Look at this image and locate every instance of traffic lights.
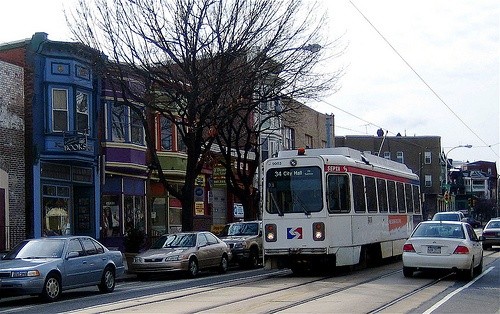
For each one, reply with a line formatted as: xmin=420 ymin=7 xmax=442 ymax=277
xmin=444 ymin=193 xmax=449 ymax=204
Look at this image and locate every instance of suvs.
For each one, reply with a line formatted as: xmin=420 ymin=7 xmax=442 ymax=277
xmin=427 ymin=211 xmax=466 ymax=222
xmin=217 ymin=220 xmax=263 ymax=268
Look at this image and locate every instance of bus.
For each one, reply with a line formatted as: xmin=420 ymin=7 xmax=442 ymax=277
xmin=260 ymin=132 xmax=424 ymax=276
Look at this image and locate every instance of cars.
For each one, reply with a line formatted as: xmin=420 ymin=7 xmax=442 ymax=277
xmin=482 ymin=219 xmax=500 ymax=250
xmin=465 ymin=217 xmax=482 ymax=229
xmin=402 ymin=221 xmax=486 ymax=281
xmin=131 ymin=231 xmax=232 ymax=281
xmin=0 ymin=235 xmax=126 ymax=303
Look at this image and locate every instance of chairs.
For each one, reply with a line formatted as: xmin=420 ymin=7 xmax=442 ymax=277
xmin=429 ymin=228 xmax=439 ymax=235
xmin=452 ymin=230 xmax=461 ymax=237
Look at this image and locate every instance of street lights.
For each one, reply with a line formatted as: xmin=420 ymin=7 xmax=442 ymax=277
xmin=445 ymin=144 xmax=473 ymax=211
xmin=251 ymin=44 xmax=323 ymax=189
